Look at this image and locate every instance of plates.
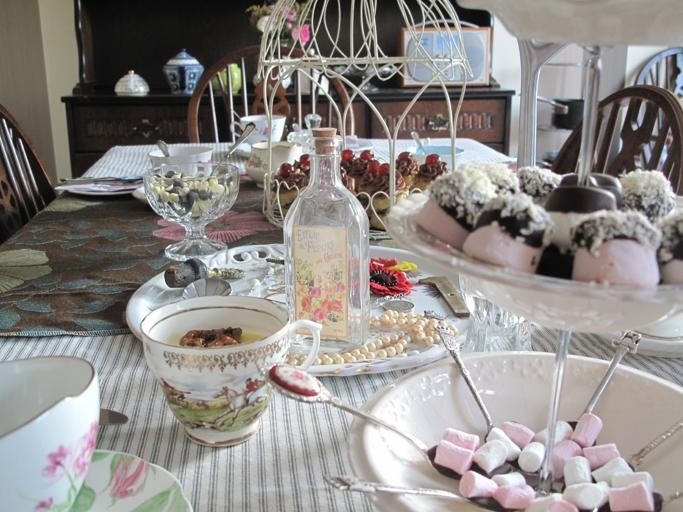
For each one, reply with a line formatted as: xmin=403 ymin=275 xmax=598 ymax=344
xmin=132 ymin=187 xmax=146 ymax=202
xmin=62 ymin=189 xmax=128 ymax=199
xmin=73 ymin=449 xmax=194 ymax=512
xmin=119 ymin=241 xmax=471 ymax=380
xmin=344 ymin=138 xmax=372 ymax=154
xmin=226 ymin=144 xmax=252 ymax=156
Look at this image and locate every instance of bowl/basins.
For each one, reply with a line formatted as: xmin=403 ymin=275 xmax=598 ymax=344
xmin=0 ymin=354 xmax=103 ymax=512
xmin=407 ymin=145 xmax=466 ymax=169
xmin=337 ymin=350 xmax=682 ymax=511
xmin=245 ymin=141 xmax=303 ymax=190
xmin=386 ymin=186 xmax=683 ymax=332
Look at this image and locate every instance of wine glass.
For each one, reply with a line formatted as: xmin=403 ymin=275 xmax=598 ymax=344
xmin=141 ymin=160 xmax=244 ymax=259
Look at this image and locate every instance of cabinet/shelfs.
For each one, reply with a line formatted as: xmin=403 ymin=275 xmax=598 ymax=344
xmin=59 ymin=93 xmax=214 ymax=177
xmin=214 ymin=93 xmax=370 ymax=141
xmin=370 ymin=90 xmax=518 ymax=157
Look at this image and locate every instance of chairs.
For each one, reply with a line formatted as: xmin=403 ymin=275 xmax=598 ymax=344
xmin=630 ymin=44 xmax=683 ymax=172
xmin=186 ymin=40 xmax=357 ymax=145
xmin=0 ymin=103 xmax=57 ymax=241
xmin=549 ymin=83 xmax=683 ymax=201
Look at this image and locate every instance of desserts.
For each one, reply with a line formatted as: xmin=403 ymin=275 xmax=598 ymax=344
xmin=268 ymin=149 xmax=683 ymax=287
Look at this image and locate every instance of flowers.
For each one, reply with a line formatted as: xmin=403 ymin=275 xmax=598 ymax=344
xmin=249 ymin=0 xmax=320 ymax=59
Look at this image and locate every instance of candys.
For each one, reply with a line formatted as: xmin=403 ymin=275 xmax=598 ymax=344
xmin=434 ymin=413 xmax=656 ymax=512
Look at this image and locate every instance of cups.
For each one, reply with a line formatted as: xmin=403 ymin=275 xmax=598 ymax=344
xmin=227 ymin=114 xmax=287 ymax=144
xmin=139 ymin=295 xmax=321 ymax=448
xmin=147 ymin=147 xmax=214 ymax=173
xmin=212 ymin=62 xmax=243 ymax=95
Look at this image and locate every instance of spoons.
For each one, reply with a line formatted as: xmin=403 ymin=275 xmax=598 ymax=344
xmin=434 ymin=326 xmax=541 ymax=476
xmin=257 ymin=365 xmax=484 ymax=479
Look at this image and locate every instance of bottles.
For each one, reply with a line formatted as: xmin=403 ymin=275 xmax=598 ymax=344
xmin=114 ymin=69 xmax=152 ymax=97
xmin=163 ymin=48 xmax=204 ymax=95
xmin=296 ymin=48 xmax=330 ymax=95
xmin=282 ymin=126 xmax=374 ymax=348
